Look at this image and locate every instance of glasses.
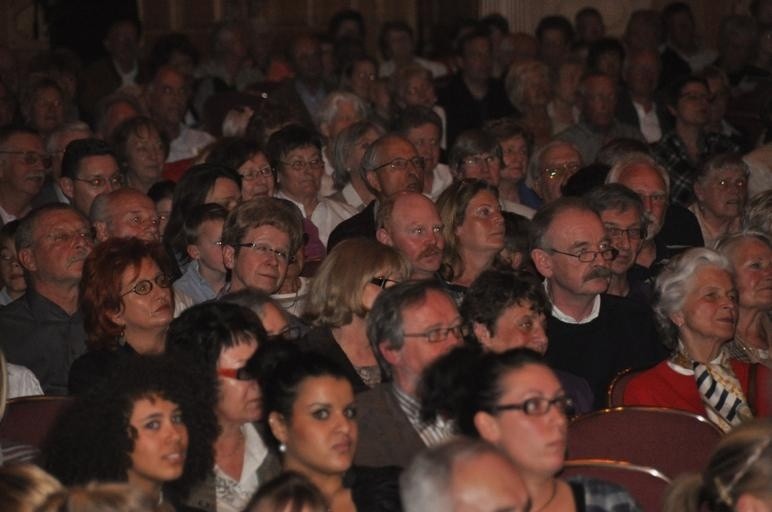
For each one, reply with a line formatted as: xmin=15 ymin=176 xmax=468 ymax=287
xmin=605 ymin=226 xmax=646 ymax=240
xmin=373 ymin=157 xmax=423 ymax=172
xmin=75 ymin=174 xmax=123 ymax=186
xmin=279 ymin=159 xmax=324 ymax=172
xmin=552 ymin=246 xmax=616 ymax=261
xmin=369 ymin=277 xmax=398 ymax=289
xmin=120 ymin=274 xmax=171 ymax=297
xmin=241 ymin=243 xmax=289 ymax=259
xmin=404 ymin=325 xmax=469 ymax=342
xmin=235 ymin=168 xmax=274 ymax=180
xmin=1 ymin=150 xmax=52 ymax=168
xmin=498 ymin=395 xmax=573 ymax=415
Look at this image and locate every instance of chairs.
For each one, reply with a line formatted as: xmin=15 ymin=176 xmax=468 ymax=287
xmin=605 ymin=364 xmax=656 ymax=405
xmin=561 ymin=404 xmax=725 ymax=487
xmin=2 ymin=392 xmax=82 ymax=465
xmin=553 ymin=457 xmax=676 ymax=512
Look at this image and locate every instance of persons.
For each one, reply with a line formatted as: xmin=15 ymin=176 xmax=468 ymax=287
xmin=0 ymin=1 xmax=772 ymax=511
